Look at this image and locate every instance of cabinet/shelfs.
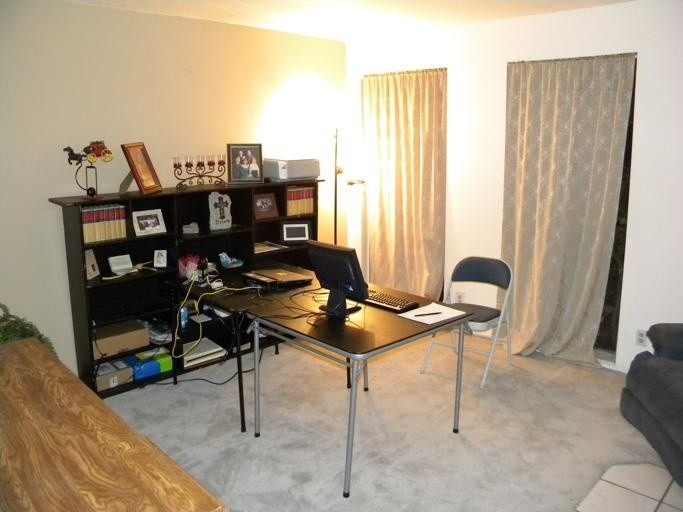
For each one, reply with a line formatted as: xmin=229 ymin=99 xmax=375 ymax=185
xmin=175 ymin=186 xmax=253 ymax=376
xmin=78 ymin=193 xmax=178 ymax=397
xmin=252 ymin=180 xmax=318 ymax=350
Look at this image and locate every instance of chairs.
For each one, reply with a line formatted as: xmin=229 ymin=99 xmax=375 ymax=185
xmin=420 ymin=255 xmax=515 ymax=391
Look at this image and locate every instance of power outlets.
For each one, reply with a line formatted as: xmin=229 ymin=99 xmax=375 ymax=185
xmin=635 ymin=328 xmax=647 ymax=347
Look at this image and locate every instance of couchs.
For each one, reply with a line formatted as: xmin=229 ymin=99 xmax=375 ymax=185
xmin=618 ymin=322 xmax=683 ymax=490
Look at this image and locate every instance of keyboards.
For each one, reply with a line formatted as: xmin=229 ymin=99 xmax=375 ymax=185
xmin=363 ymin=289 xmax=419 ymax=313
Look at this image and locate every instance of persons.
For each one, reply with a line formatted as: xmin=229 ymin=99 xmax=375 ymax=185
xmin=235 ymin=149 xmax=260 ymax=179
xmin=157 ymin=252 xmax=165 ymax=265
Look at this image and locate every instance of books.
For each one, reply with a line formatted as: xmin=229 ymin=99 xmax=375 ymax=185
xmin=82 ymin=206 xmax=129 ymax=244
xmin=286 ymin=187 xmax=315 ymax=215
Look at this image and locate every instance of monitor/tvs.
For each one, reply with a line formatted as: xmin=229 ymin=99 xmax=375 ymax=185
xmin=305 ymin=238 xmax=369 ymax=318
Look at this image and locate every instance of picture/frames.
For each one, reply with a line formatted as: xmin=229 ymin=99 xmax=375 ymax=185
xmin=251 ymin=192 xmax=280 ymax=220
xmin=226 ymin=143 xmax=264 ymax=185
xmin=279 ymin=221 xmax=313 ymax=245
xmin=132 ymin=208 xmax=168 ymax=237
xmin=120 ymin=142 xmax=163 ymax=197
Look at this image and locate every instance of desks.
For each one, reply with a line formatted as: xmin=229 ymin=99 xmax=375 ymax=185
xmin=170 ymin=259 xmax=328 ymax=434
xmin=245 ymin=281 xmax=476 ymax=498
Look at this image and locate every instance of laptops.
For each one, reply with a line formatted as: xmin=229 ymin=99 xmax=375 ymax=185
xmin=251 ymin=267 xmax=313 ymax=291
